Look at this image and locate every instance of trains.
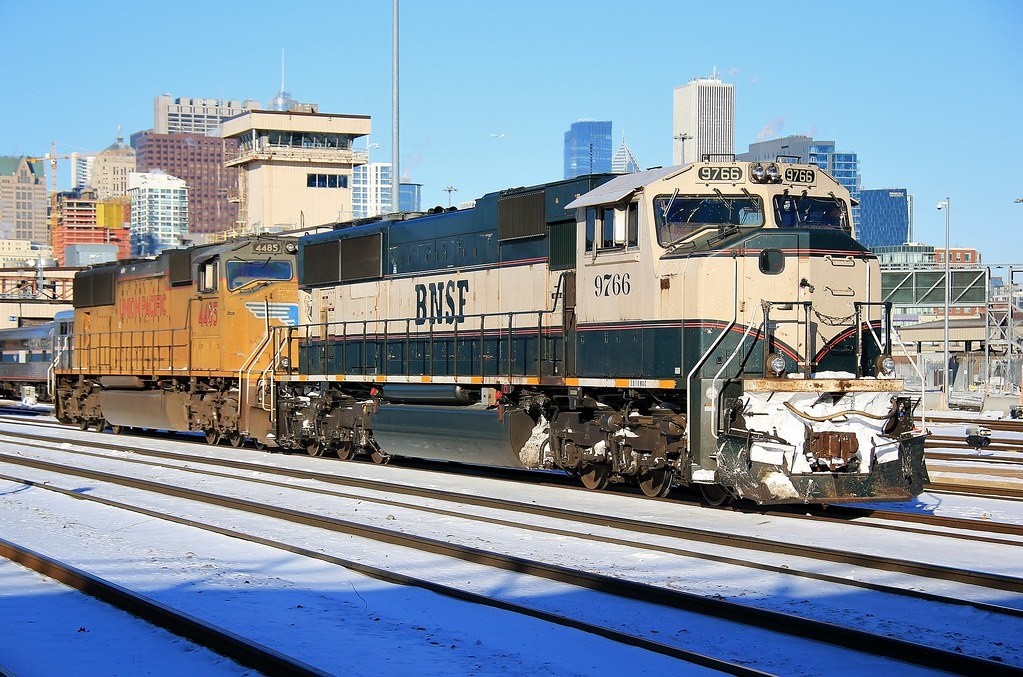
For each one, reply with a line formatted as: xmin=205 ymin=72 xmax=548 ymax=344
xmin=46 ymin=149 xmax=934 ymax=523
xmin=0 ymin=308 xmax=75 ymax=403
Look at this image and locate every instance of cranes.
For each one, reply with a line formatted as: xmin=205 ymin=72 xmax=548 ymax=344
xmin=24 ymin=142 xmax=136 ymax=267
